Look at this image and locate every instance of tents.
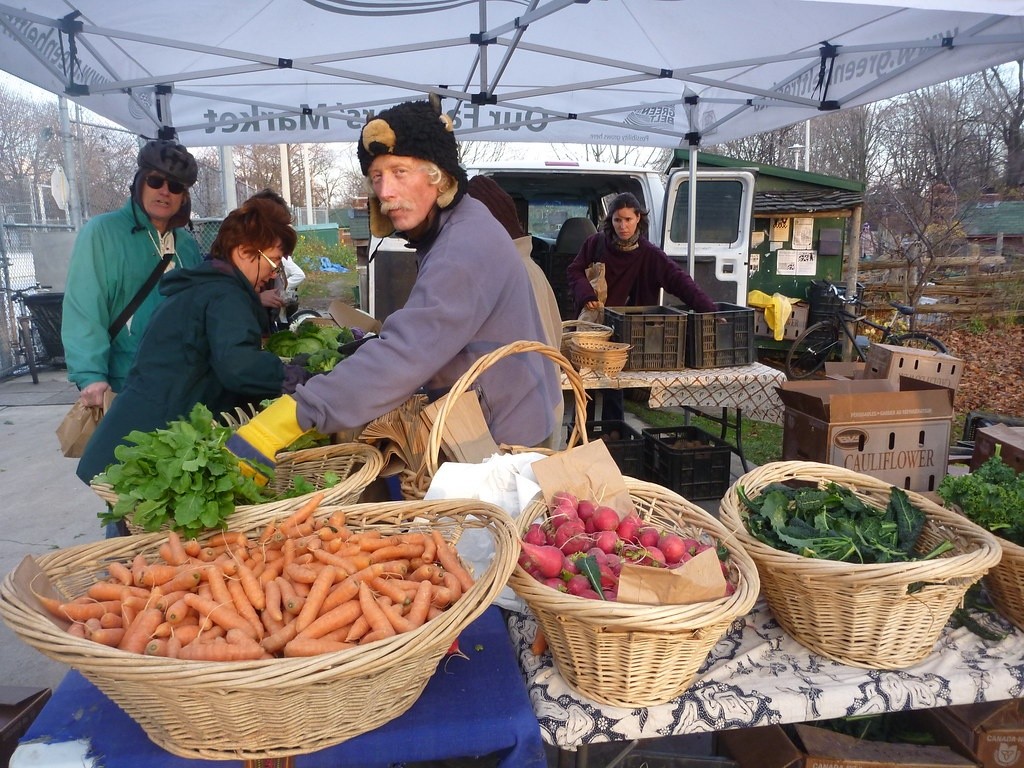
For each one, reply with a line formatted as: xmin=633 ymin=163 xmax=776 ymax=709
xmin=0 ymin=0 xmax=1024 ymax=317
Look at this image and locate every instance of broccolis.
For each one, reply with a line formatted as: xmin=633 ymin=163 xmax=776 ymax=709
xmin=302 ymin=349 xmax=343 ymax=371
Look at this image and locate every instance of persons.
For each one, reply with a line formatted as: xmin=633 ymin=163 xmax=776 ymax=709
xmin=567 ymin=193 xmax=727 ymax=432
xmin=61 ymin=101 xmax=564 ymax=576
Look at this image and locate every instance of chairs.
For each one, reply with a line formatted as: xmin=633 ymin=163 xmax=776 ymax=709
xmin=556 ymin=217 xmax=599 ymax=252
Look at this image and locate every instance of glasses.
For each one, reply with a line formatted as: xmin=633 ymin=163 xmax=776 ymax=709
xmin=257 ymin=248 xmax=281 ymax=277
xmin=144 ymin=176 xmax=185 ymax=195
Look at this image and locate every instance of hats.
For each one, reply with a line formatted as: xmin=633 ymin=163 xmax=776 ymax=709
xmin=465 ymin=176 xmax=524 ymax=239
xmin=357 ymin=100 xmax=467 ymax=209
xmin=137 ymin=141 xmax=198 ymax=186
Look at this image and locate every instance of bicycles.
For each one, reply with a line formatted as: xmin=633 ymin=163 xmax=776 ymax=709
xmin=0 ymin=282 xmax=54 ymax=385
xmin=785 ymin=277 xmax=950 ymax=383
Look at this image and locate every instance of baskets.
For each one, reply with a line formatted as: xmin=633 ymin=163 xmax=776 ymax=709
xmin=3 ymin=462 xmax=1024 ymax=760
xmin=91 ymin=442 xmax=382 ymax=536
xmin=400 ymin=344 xmax=589 ymax=502
xmin=560 ymin=319 xmax=634 ymax=377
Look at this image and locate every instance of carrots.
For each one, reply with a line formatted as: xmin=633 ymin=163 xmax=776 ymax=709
xmin=532 ymin=625 xmax=549 ymax=655
xmin=28 ymin=492 xmax=477 ymax=662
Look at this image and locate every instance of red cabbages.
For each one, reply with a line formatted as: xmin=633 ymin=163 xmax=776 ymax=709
xmin=348 ymin=326 xmax=365 ymax=341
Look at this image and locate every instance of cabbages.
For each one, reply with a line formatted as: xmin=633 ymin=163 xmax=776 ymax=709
xmin=267 ymin=319 xmax=355 ymax=357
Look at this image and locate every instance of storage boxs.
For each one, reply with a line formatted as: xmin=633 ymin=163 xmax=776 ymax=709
xmin=807 ymin=280 xmax=865 ymax=340
xmin=349 ymin=192 xmax=370 ymax=240
xmin=304 ymin=300 xmax=383 ymax=335
xmin=669 ymin=301 xmax=756 ymax=369
xmin=969 ymin=423 xmax=1024 ymax=477
xmin=749 ymin=301 xmax=809 ymax=340
xmin=772 ymin=343 xmax=967 ymax=505
xmin=642 ymin=425 xmax=731 ymax=500
xmin=957 ymin=411 xmax=1024 ymax=448
xmin=712 ymin=698 xmax=1023 ymax=768
xmin=530 ymin=250 xmax=578 ymax=303
xmin=0 ymin=685 xmax=52 ymax=767
xmin=566 ymin=419 xmax=644 ymax=477
xmin=604 ymin=306 xmax=688 ymax=372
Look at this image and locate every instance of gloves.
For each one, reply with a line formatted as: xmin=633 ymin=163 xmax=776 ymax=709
xmin=225 ymin=397 xmax=314 ymax=485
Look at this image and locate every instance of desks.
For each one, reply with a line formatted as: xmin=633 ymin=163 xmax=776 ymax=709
xmin=8 ymin=605 xmax=551 ymax=767
xmin=560 ymin=361 xmax=788 ymax=474
xmin=507 ymin=589 xmax=1023 ymax=768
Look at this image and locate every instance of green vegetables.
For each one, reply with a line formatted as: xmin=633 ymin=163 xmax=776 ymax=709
xmin=935 ymin=442 xmax=1024 ymax=550
xmin=737 ymin=481 xmax=954 ymax=594
xmin=86 ymin=400 xmax=341 ymax=541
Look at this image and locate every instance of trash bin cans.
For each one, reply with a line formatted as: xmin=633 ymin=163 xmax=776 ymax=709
xmin=25 ymin=291 xmax=64 ymax=368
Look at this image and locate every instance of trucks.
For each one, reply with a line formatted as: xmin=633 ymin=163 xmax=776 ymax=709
xmin=365 ymin=158 xmax=760 ymax=325
